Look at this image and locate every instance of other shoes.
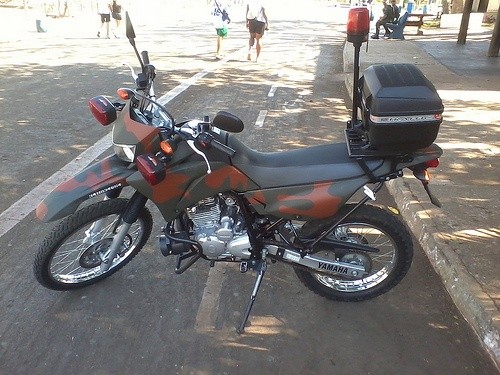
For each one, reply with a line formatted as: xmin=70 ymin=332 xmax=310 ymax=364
xmin=384 ymin=32 xmax=391 ymax=37
xmin=97 ymin=31 xmax=100 ymax=37
xmin=371 ymin=35 xmax=379 ymax=39
xmin=216 ymin=55 xmax=221 ymax=60
xmin=247 ymin=52 xmax=252 ymax=60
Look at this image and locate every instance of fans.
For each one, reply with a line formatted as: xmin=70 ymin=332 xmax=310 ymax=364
xmin=356 ymin=63 xmax=445 ymax=151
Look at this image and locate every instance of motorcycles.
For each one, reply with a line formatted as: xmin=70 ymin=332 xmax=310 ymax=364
xmin=32 ymin=10 xmax=445 ymax=335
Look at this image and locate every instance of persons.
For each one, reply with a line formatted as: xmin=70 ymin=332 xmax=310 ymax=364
xmin=245 ymin=0 xmax=269 ymax=62
xmin=96 ymin=0 xmax=113 ymax=39
xmin=210 ymin=0 xmax=230 ymax=60
xmin=111 ymin=0 xmax=123 ymax=39
xmin=370 ymin=0 xmax=400 ymax=39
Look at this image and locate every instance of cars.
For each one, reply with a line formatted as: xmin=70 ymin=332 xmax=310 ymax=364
xmin=328 ymin=0 xmax=375 ymax=21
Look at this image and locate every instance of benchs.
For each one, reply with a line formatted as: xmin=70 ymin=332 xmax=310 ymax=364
xmin=386 ymin=12 xmax=409 ymax=40
